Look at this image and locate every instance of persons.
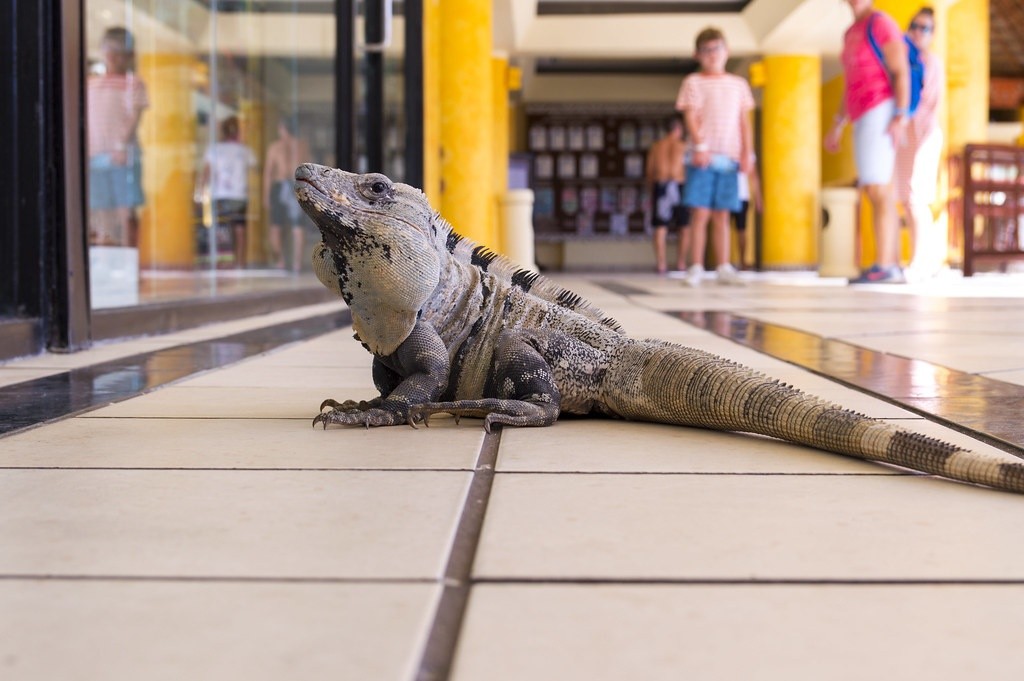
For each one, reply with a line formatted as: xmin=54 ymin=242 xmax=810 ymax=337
xmin=645 ymin=118 xmax=764 ymax=279
xmin=676 ymin=29 xmax=759 ymax=286
xmin=85 ymin=26 xmax=146 ymax=245
xmin=194 ymin=115 xmax=319 ymax=273
xmin=822 ymin=0 xmax=941 ymax=286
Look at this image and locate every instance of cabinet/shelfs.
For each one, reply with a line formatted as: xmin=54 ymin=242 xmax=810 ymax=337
xmin=947 ymin=143 xmax=1024 ymax=276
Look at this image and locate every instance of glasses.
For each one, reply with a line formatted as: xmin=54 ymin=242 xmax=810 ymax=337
xmin=909 ymin=21 xmax=934 ymax=35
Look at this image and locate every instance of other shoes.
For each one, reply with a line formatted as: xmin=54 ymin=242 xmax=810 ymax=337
xmin=717 ymin=264 xmax=748 ymax=286
xmin=683 ymin=264 xmax=704 ymax=287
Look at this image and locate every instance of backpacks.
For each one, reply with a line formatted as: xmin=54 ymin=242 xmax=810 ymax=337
xmin=865 ymin=13 xmax=923 ymax=114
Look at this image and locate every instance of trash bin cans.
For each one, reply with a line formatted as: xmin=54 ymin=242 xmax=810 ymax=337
xmin=818 ymin=187 xmax=858 ymax=278
xmin=506 ymin=188 xmax=540 ymax=277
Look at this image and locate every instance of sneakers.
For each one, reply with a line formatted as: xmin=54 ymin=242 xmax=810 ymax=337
xmin=848 ymin=264 xmax=907 ymax=284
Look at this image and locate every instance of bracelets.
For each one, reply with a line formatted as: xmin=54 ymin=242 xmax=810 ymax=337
xmin=834 ymin=113 xmax=848 ymax=126
xmin=690 ymin=142 xmax=708 ymax=152
xmin=112 ymin=138 xmax=128 ymax=152
xmin=744 ymin=150 xmax=757 ymax=163
